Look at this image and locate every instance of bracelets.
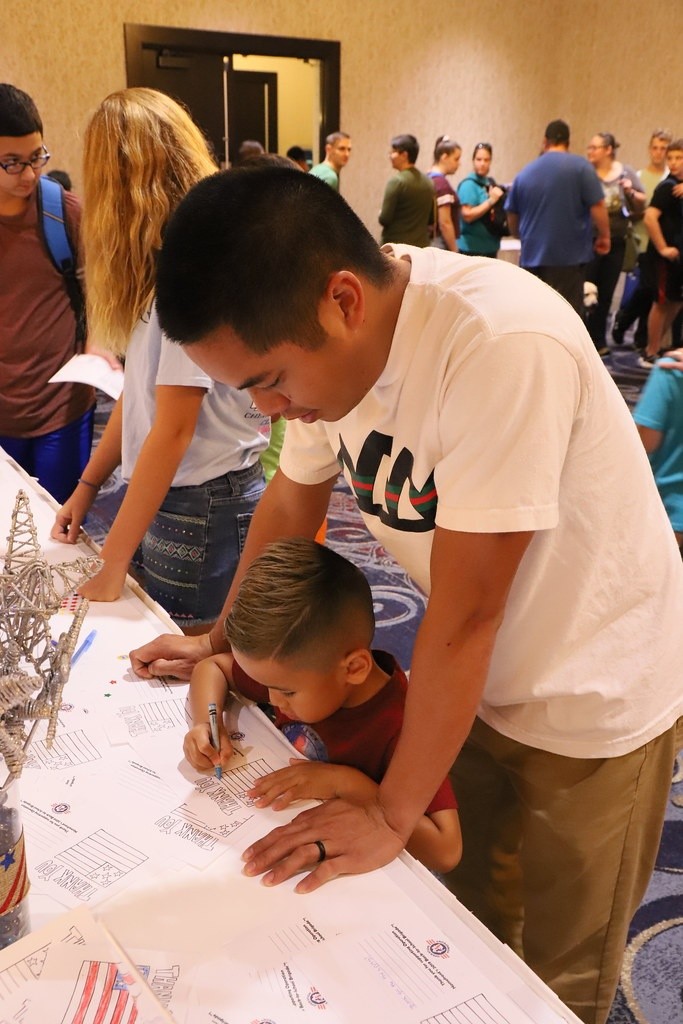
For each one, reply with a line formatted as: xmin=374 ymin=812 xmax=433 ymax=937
xmin=78 ymin=476 xmax=102 ymax=494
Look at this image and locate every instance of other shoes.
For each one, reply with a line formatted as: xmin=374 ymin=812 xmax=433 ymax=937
xmin=597 ymin=346 xmax=611 ymax=359
xmin=638 ymin=347 xmax=668 ymax=368
xmin=612 ymin=311 xmax=625 ymax=343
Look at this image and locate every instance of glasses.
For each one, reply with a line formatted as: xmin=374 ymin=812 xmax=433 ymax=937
xmin=0 ymin=146 xmax=51 ymax=175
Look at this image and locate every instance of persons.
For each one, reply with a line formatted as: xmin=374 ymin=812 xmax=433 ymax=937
xmin=633 ymin=352 xmax=683 ymax=546
xmin=131 ymin=164 xmax=681 ymax=1021
xmin=234 ymin=115 xmax=682 ymax=372
xmin=183 ymin=535 xmax=463 ymax=876
xmin=52 ymin=88 xmax=267 ymax=630
xmin=1 ymin=83 xmax=94 ymax=510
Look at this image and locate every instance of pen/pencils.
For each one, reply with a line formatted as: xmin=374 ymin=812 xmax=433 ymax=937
xmin=70 ymin=629 xmax=98 ymax=666
xmin=208 ymin=703 xmax=221 ymax=780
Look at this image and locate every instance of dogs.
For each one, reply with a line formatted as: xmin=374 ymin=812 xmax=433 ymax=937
xmin=583 ymin=282 xmax=599 ymax=317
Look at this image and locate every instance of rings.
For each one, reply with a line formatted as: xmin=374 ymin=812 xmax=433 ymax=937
xmin=312 ymin=840 xmax=327 ymax=864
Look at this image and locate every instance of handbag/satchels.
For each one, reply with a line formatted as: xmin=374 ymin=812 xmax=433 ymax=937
xmin=621 ymin=226 xmax=642 ymax=272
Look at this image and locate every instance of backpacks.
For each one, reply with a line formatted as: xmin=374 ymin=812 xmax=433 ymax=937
xmin=458 ymin=177 xmax=511 ymax=237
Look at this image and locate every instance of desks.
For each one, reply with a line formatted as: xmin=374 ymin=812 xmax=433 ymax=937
xmin=0 ymin=445 xmax=583 ymax=1024
